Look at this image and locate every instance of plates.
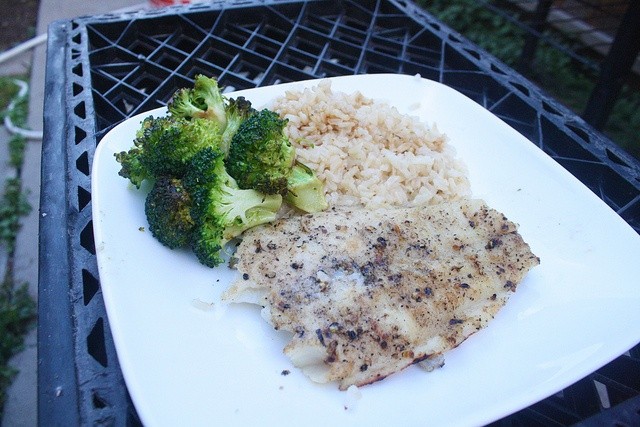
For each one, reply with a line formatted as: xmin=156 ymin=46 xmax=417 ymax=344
xmin=91 ymin=73 xmax=640 ymax=427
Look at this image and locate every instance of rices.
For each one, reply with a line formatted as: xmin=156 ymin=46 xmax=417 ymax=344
xmin=269 ymin=80 xmax=470 ymax=206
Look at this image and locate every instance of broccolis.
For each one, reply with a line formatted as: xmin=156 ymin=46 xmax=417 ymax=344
xmin=113 ymin=73 xmax=326 ymax=270
